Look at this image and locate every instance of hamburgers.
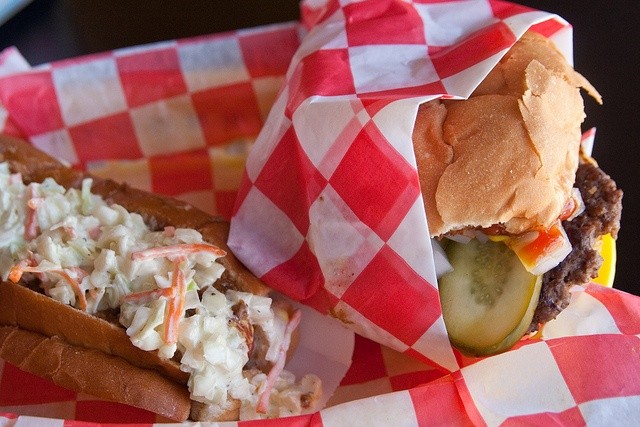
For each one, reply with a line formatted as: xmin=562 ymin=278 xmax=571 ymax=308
xmin=412 ymin=30 xmax=622 ymax=359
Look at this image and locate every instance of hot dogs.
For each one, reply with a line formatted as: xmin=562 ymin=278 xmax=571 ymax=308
xmin=2 ymin=131 xmax=298 ymax=422
